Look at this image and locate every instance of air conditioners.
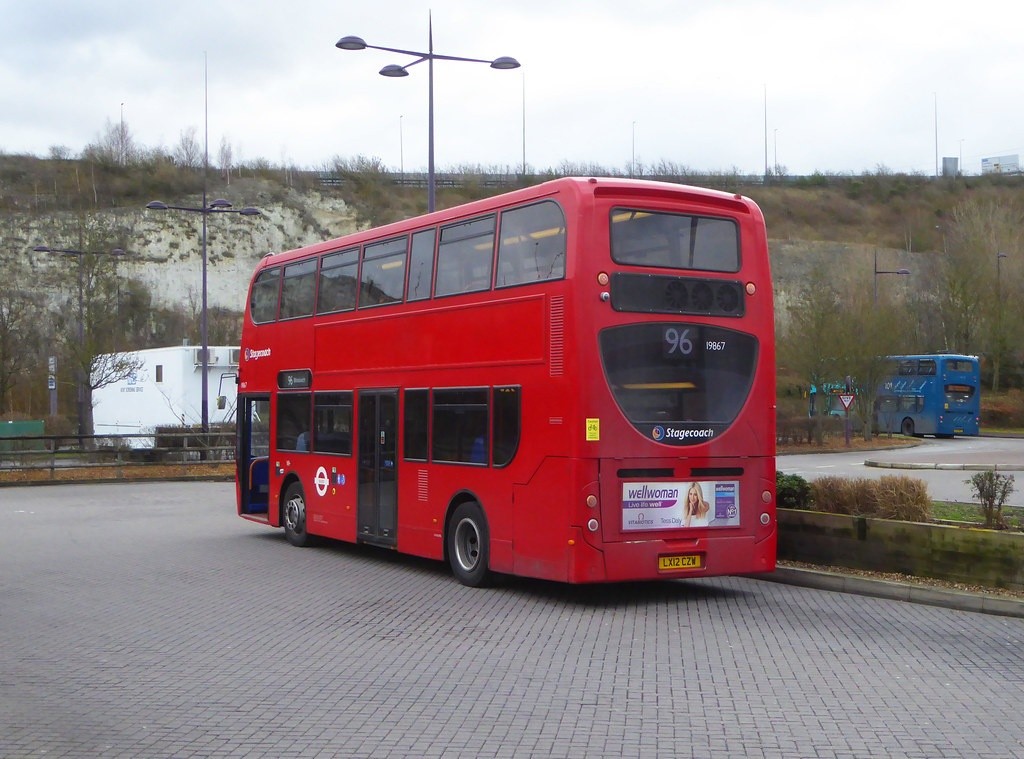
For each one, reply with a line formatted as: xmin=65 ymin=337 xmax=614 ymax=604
xmin=228 ymin=348 xmax=241 ymax=365
xmin=193 ymin=347 xmax=216 ymax=365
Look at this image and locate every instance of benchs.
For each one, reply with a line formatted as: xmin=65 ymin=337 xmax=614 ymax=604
xmin=154 ymin=427 xmax=221 ymax=460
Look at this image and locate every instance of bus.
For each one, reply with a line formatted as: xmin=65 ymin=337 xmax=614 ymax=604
xmin=216 ymin=176 xmax=779 ymax=594
xmin=807 ymin=352 xmax=983 ymax=438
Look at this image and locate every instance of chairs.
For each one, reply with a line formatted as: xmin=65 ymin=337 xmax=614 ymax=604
xmin=296 ymin=431 xmax=311 ymax=451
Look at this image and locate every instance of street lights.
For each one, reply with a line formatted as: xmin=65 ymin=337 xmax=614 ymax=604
xmin=144 ymin=187 xmax=261 ymax=461
xmin=119 ymin=101 xmax=125 ymax=165
xmin=872 ymin=247 xmax=911 ymax=306
xmin=996 ymin=249 xmax=1007 ymax=296
xmin=632 ymin=120 xmax=635 ymax=178
xmin=334 ymin=8 xmax=524 ymax=213
xmin=400 ymin=114 xmax=405 ymax=184
xmin=33 ymin=226 xmax=130 ymax=450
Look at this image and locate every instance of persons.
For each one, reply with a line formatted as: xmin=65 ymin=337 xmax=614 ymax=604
xmin=682 ymin=482 xmax=710 ymax=527
xmin=296 ymin=419 xmax=311 ymax=452
xmin=471 ymin=424 xmax=494 ymax=464
